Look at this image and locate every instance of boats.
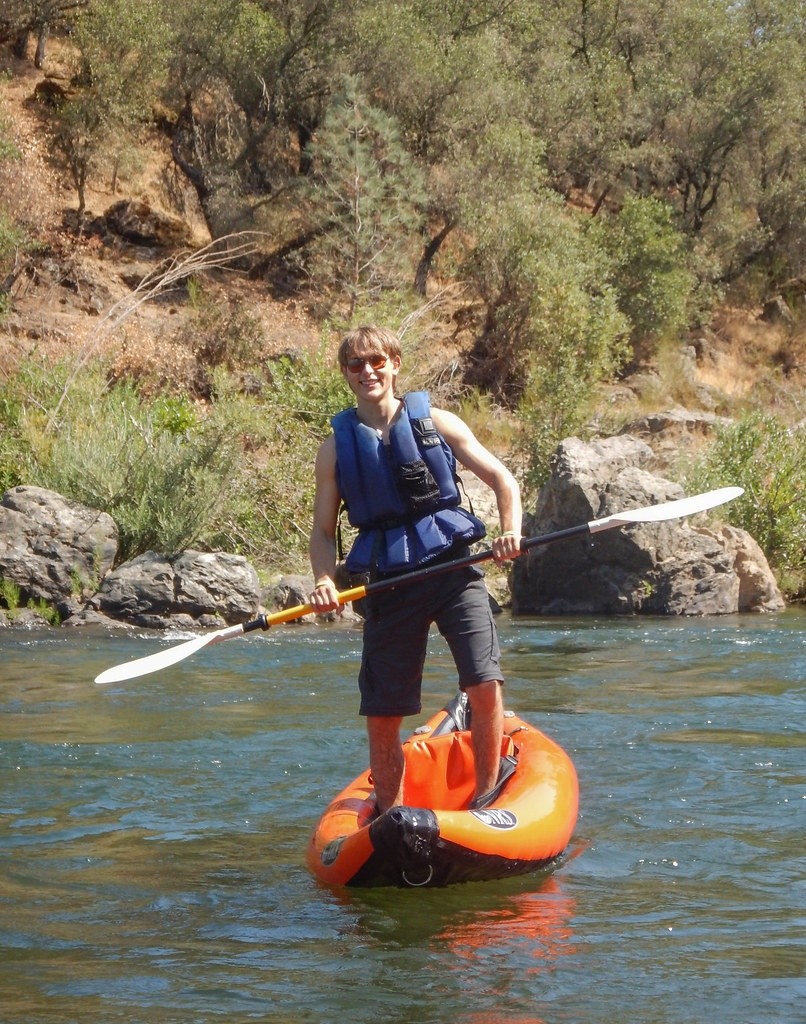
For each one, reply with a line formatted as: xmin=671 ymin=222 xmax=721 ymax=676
xmin=305 ymin=685 xmax=580 ymax=889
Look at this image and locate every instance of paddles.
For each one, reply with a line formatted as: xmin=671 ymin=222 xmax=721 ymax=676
xmin=92 ymin=486 xmax=745 ymax=687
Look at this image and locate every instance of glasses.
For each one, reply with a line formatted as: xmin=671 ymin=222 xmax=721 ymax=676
xmin=343 ymin=355 xmax=391 ymax=373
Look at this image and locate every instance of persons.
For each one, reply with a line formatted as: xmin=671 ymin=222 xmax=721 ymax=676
xmin=309 ymin=326 xmax=523 ymax=816
